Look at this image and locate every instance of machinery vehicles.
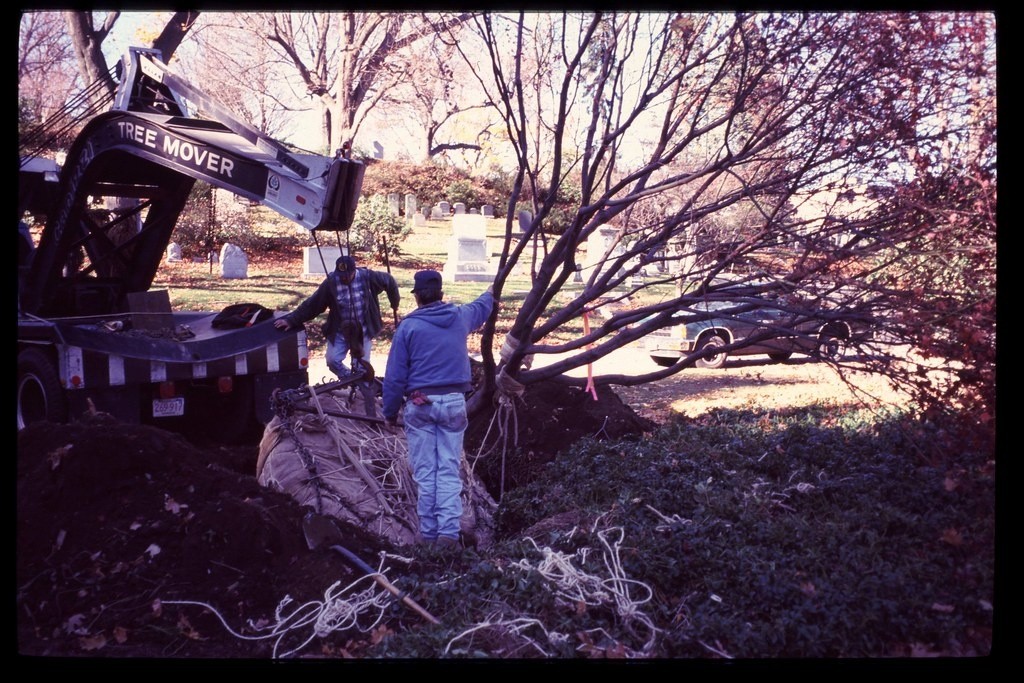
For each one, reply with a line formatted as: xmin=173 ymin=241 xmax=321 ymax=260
xmin=21 ymin=45 xmax=368 ymax=452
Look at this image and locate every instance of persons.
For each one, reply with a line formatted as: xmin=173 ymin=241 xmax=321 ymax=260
xmin=385 ymin=269 xmax=499 ymax=555
xmin=274 ymin=257 xmax=400 ymax=385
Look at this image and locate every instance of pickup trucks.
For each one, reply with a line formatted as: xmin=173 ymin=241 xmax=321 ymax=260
xmin=646 ymin=279 xmax=876 ymax=368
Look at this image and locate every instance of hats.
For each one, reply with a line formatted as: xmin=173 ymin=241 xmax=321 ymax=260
xmin=410 ymin=270 xmax=442 ymax=293
xmin=334 ymin=256 xmax=354 ymax=272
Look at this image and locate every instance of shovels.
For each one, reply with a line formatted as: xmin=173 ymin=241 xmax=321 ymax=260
xmin=302 ymin=512 xmax=441 ymax=625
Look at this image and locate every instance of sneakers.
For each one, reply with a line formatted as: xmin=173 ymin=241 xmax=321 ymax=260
xmin=428 ymin=538 xmax=462 ymax=554
